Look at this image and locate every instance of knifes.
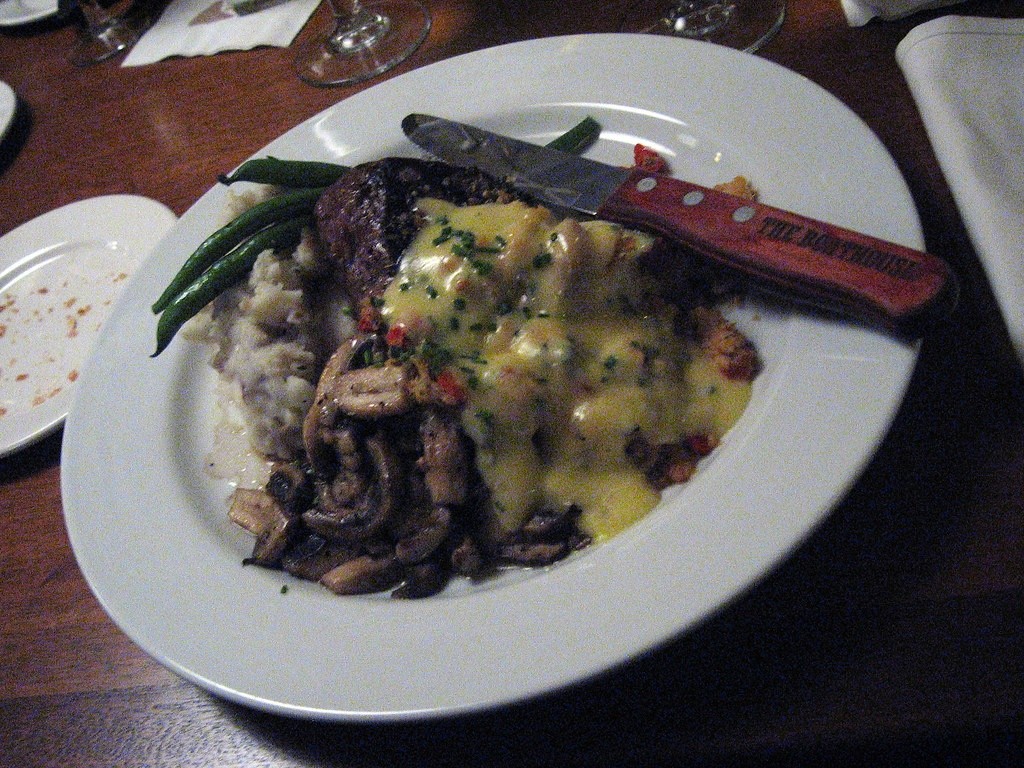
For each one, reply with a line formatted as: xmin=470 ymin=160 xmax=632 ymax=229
xmin=190 ymin=1 xmax=288 ymax=26
xmin=402 ymin=113 xmax=957 ymax=335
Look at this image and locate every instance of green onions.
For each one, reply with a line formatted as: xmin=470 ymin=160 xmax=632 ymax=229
xmin=336 ymin=217 xmax=617 ymax=432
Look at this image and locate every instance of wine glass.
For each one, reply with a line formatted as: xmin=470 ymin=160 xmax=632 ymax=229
xmin=293 ymin=0 xmax=430 ymax=87
xmin=56 ymin=1 xmax=149 ymax=69
xmin=617 ymin=2 xmax=786 ymax=53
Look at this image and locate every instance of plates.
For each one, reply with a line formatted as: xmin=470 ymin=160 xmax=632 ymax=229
xmin=1 ymin=1 xmax=57 ymax=26
xmin=1 ymin=194 xmax=178 ymax=459
xmin=60 ymin=32 xmax=925 ymax=722
xmin=1 ymin=82 xmax=18 ymax=141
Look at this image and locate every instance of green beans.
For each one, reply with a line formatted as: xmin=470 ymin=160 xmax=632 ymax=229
xmin=148 ymin=118 xmax=598 ymax=358
